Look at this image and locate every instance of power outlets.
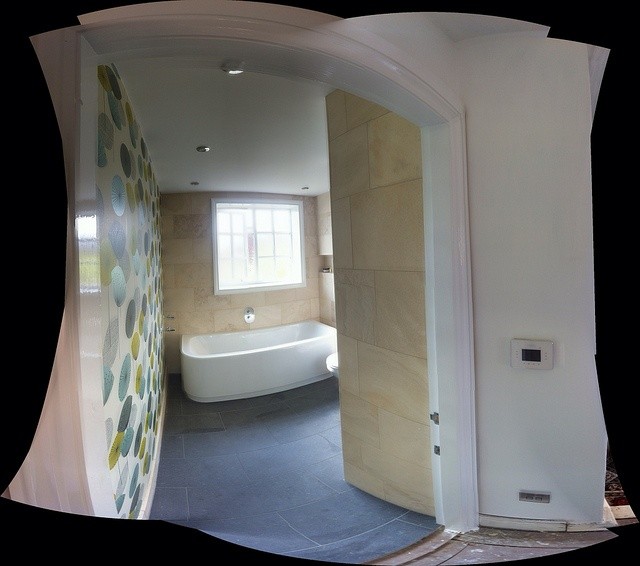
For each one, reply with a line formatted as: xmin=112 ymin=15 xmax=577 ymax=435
xmin=519 ymin=491 xmax=552 ymax=504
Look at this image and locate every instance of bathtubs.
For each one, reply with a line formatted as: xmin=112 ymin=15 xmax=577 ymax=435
xmin=179 ymin=320 xmax=337 ymax=405
